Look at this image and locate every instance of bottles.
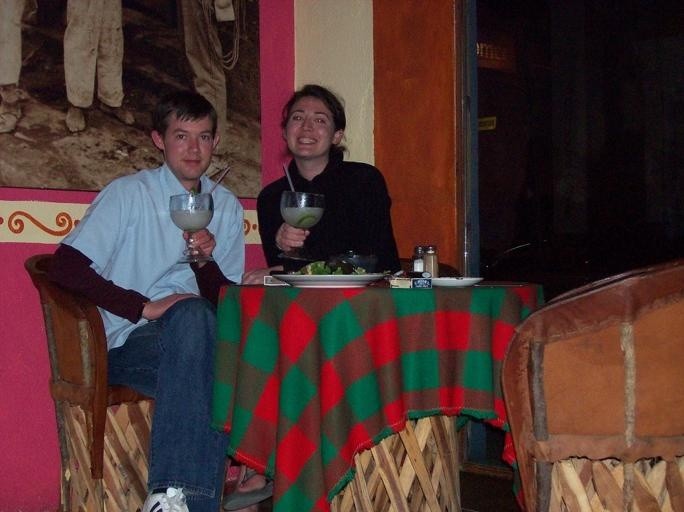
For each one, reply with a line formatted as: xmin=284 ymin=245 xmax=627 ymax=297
xmin=410 ymin=246 xmax=424 ymax=278
xmin=422 ymin=246 xmax=439 ymax=278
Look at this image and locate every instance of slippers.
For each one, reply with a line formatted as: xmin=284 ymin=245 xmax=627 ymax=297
xmin=223 ymin=470 xmax=274 ymax=510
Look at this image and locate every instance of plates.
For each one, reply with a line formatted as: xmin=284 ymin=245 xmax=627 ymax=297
xmin=272 ymin=273 xmax=386 ymax=288
xmin=383 ymin=270 xmax=484 ymax=288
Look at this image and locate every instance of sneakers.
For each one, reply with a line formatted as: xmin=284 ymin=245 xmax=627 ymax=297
xmin=142 ymin=488 xmax=189 ymax=512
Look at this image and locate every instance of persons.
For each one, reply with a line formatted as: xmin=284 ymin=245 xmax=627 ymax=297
xmin=215 ymin=82 xmax=401 ymax=511
xmin=60 ymin=0 xmax=134 ymax=132
xmin=173 ymin=0 xmax=240 ymax=152
xmin=0 ymin=1 xmax=30 ymax=102
xmin=43 ymin=90 xmax=249 ymax=511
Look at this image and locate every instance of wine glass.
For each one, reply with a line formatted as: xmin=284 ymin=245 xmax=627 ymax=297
xmin=168 ymin=193 xmax=215 ymax=263
xmin=277 ymin=191 xmax=325 ymax=263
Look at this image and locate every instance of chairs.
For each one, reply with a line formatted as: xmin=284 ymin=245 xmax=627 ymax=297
xmin=501 ymin=262 xmax=683 ymax=510
xmin=23 ymin=254 xmax=155 ymax=512
